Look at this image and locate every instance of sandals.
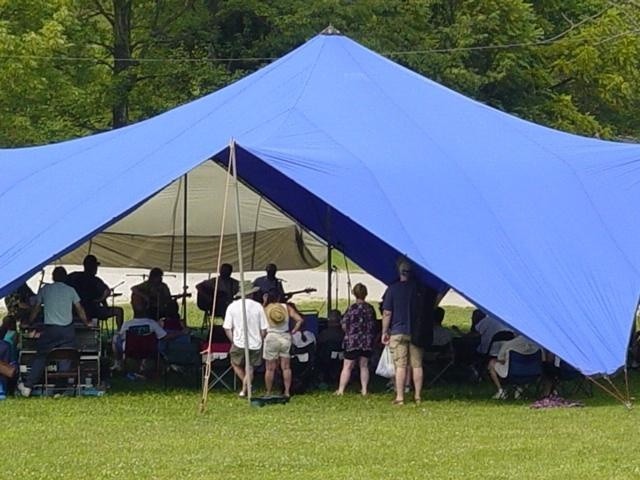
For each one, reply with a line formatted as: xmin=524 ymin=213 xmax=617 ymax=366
xmin=415 ymin=397 xmax=421 ymax=403
xmin=393 ymin=399 xmax=404 ymax=405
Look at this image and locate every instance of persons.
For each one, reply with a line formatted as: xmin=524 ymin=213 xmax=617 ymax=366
xmin=223 ymin=280 xmax=270 ymax=397
xmin=290 ymin=312 xmax=316 ymax=382
xmin=0 ymin=254 xmax=202 ymax=399
xmin=430 ymin=304 xmax=587 ymax=401
xmin=196 ymin=262 xmax=240 ymax=318
xmin=332 ymin=282 xmax=377 ymax=396
xmin=380 ymin=259 xmax=434 ymax=406
xmin=317 ymin=309 xmax=347 ymax=384
xmin=206 ymin=325 xmax=230 ymax=345
xmin=251 ymin=262 xmax=285 ymax=306
xmin=261 ymin=285 xmax=306 ymax=401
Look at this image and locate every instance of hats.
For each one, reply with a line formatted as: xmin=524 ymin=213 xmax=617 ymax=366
xmin=232 ymin=280 xmax=261 ymax=299
xmin=83 ymin=254 xmax=100 ymax=265
xmin=399 ymin=261 xmax=411 ymax=273
xmin=264 ymin=303 xmax=289 ymax=326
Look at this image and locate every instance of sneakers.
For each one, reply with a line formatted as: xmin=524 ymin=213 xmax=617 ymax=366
xmin=514 ymin=388 xmax=524 ymax=399
xmin=239 ymin=390 xmax=246 ymax=396
xmin=243 ymin=375 xmax=247 ymax=394
xmin=54 ymin=392 xmax=64 ymax=399
xmin=491 ymin=390 xmax=509 ymax=400
xmin=110 ymin=361 xmax=122 ymax=371
xmin=18 ymin=382 xmax=31 ymax=397
